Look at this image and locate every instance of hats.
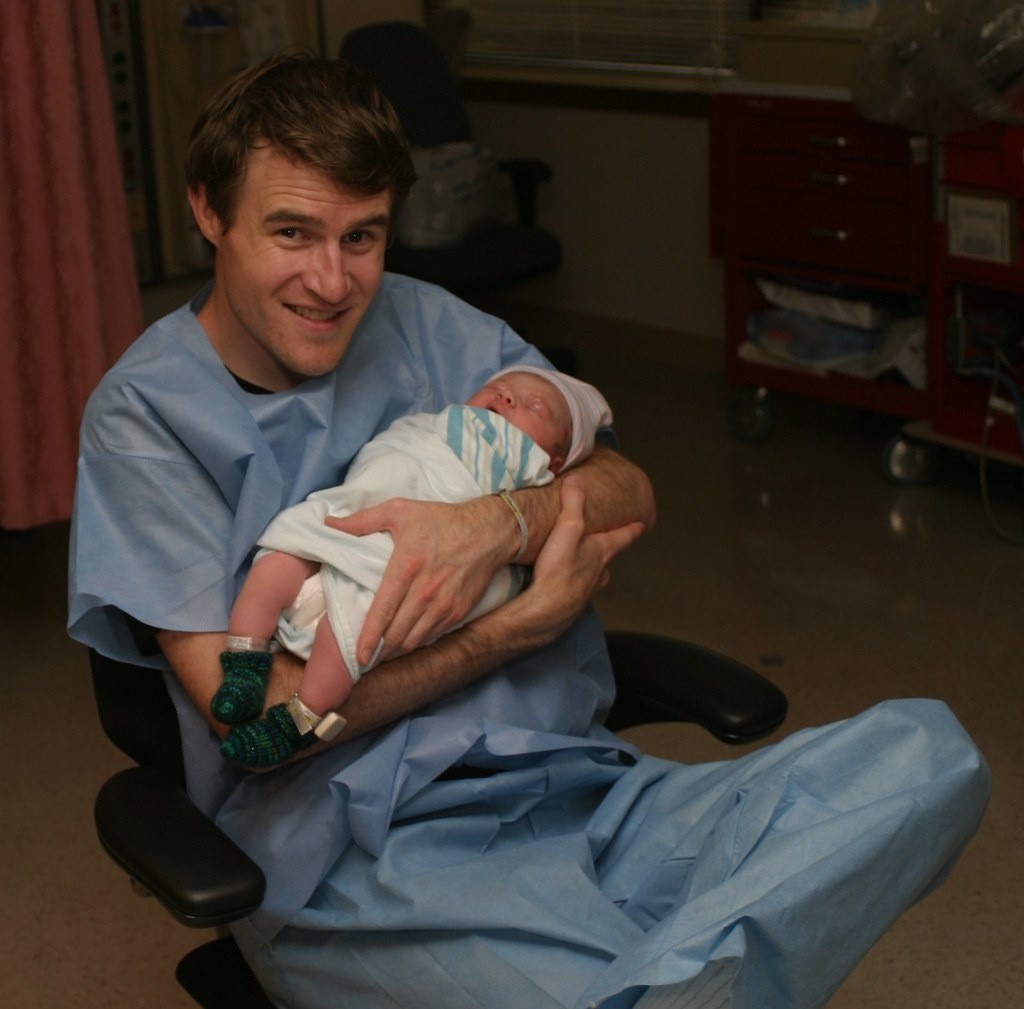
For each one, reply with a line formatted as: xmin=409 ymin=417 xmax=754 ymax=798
xmin=482 ymin=363 xmax=614 ymax=475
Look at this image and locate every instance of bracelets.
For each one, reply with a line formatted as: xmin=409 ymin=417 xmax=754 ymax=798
xmin=493 ymin=491 xmax=530 ymax=562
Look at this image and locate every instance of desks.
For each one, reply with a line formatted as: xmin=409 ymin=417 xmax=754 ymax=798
xmin=391 ymin=30 xmax=1024 ymax=465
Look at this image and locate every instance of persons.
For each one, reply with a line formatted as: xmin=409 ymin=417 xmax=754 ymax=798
xmin=68 ymin=50 xmax=994 ymax=1009
xmin=210 ymin=365 xmax=612 ymax=770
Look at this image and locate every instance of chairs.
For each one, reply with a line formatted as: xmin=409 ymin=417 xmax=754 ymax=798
xmin=339 ymin=19 xmax=576 ymax=374
xmin=91 ymin=632 xmax=790 ymax=1009
